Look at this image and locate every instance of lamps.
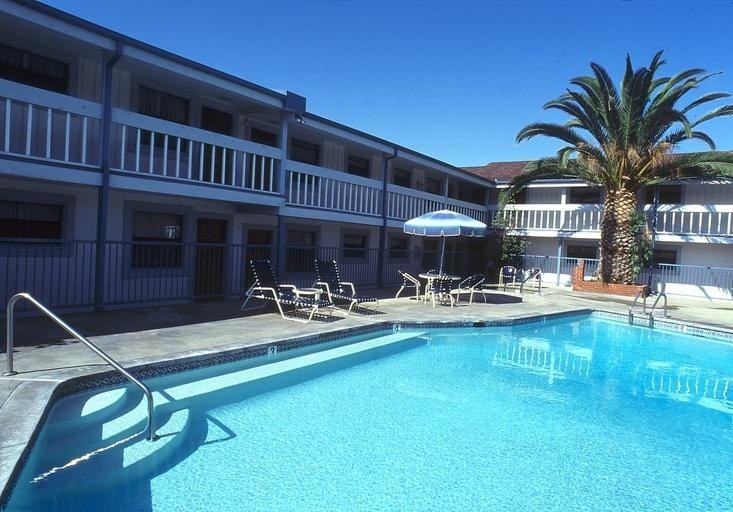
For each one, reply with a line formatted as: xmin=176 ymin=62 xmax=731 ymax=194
xmin=240 ymin=259 xmax=333 ymax=324
xmin=394 ymin=265 xmax=542 ymax=308
xmin=310 ymin=259 xmax=379 ymax=314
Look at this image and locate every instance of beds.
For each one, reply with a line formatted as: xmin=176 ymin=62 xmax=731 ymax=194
xmin=403 ymin=206 xmax=490 ymax=298
